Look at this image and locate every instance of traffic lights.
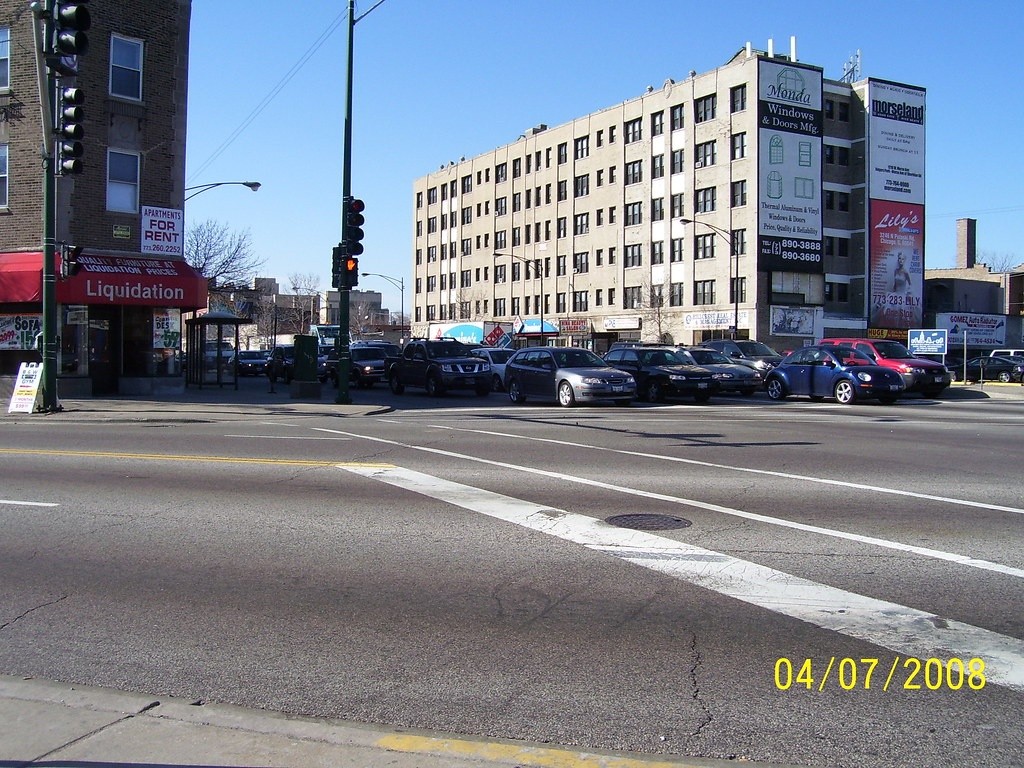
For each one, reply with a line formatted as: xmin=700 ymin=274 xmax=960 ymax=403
xmin=58 ymin=240 xmax=84 ymax=278
xmin=53 ymin=77 xmax=86 ymax=141
xmin=52 ymin=139 xmax=85 ymax=177
xmin=345 ymin=196 xmax=365 ymax=256
xmin=42 ymin=0 xmax=92 ymax=59
xmin=345 ymin=257 xmax=360 ymax=287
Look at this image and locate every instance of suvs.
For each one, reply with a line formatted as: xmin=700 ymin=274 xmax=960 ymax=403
xmin=817 ymin=334 xmax=951 ymax=398
xmin=384 ymin=336 xmax=492 ymax=399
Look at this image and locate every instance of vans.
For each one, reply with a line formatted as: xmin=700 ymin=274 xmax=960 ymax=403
xmin=990 ymin=349 xmax=1024 ymax=365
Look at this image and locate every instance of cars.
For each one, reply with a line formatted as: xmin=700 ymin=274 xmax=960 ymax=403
xmin=599 ymin=345 xmax=720 ymax=402
xmin=176 ymin=324 xmax=484 ymax=391
xmin=504 ymin=345 xmax=638 ymax=407
xmin=463 ymin=346 xmax=524 ymax=393
xmin=764 ymin=345 xmax=907 ymax=406
xmin=609 ymin=334 xmax=785 ymax=396
xmin=948 ymin=355 xmax=1024 ymax=383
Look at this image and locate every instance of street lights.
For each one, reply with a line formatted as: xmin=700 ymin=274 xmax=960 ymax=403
xmin=292 ymin=286 xmax=329 ymax=325
xmin=679 ymin=219 xmax=740 ymax=340
xmin=492 ymin=252 xmax=545 ymax=345
xmin=360 ymin=272 xmax=405 ymax=351
